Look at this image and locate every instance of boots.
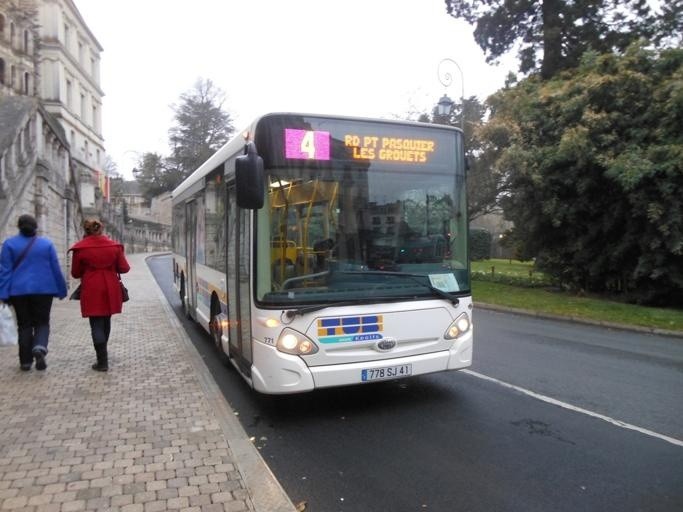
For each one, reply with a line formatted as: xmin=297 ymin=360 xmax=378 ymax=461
xmin=92 ymin=343 xmax=108 ymax=371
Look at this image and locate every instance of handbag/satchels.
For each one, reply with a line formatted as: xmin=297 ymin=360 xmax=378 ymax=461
xmin=120 ymin=282 xmax=129 ymax=302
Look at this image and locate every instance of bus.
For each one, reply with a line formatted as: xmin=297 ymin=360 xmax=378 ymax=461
xmin=167 ymin=108 xmax=475 ymax=409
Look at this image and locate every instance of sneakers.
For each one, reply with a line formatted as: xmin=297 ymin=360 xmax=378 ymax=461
xmin=34 ymin=351 xmax=46 ymax=370
xmin=21 ymin=364 xmax=31 ymax=371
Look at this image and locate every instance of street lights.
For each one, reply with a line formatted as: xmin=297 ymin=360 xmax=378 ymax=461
xmin=435 ymin=57 xmax=464 ymax=130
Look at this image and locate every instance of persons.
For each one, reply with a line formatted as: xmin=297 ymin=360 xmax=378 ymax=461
xmin=0 ymin=213 xmax=67 ymax=370
xmin=66 ymin=218 xmax=130 ymax=370
